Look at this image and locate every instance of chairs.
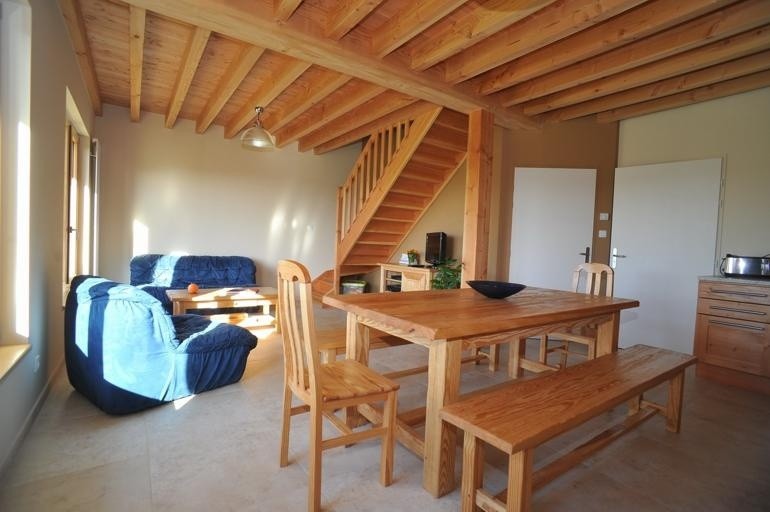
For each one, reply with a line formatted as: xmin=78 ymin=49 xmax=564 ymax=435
xmin=539 ymin=262 xmax=613 ymax=369
xmin=64 ymin=275 xmax=257 ymax=414
xmin=277 ymin=259 xmax=400 ymax=512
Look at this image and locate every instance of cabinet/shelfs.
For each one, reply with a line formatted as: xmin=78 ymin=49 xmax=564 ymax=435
xmin=694 ymin=281 xmax=770 ymax=395
xmin=380 ymin=263 xmax=433 ymax=293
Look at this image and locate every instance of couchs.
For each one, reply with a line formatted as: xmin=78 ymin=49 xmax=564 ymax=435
xmin=130 ymin=255 xmax=256 ymax=315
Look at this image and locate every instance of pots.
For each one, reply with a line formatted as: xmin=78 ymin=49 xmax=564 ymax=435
xmin=719 ymin=252 xmax=770 ymax=278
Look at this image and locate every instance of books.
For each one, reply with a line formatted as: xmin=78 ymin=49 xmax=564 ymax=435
xmin=226 ymin=288 xmax=260 ymax=295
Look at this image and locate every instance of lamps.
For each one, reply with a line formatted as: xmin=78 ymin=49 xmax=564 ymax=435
xmin=240 ymin=107 xmax=274 ymax=148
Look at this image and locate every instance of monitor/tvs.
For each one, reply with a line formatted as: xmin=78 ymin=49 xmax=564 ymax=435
xmin=425 ymin=232 xmax=447 ymax=268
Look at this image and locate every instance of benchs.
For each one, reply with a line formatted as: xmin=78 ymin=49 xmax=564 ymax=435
xmin=300 ymin=324 xmax=500 ymax=379
xmin=438 ymin=344 xmax=699 ymax=512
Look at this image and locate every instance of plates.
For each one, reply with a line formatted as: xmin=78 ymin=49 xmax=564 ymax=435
xmin=467 ymin=280 xmax=527 ymax=299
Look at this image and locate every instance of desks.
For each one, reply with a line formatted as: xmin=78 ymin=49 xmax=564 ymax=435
xmin=322 ymin=287 xmax=640 ymax=499
xmin=165 ymin=287 xmax=281 ymax=333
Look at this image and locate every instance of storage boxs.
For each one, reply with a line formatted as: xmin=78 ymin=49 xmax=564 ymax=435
xmin=341 ymin=280 xmax=368 ymax=294
